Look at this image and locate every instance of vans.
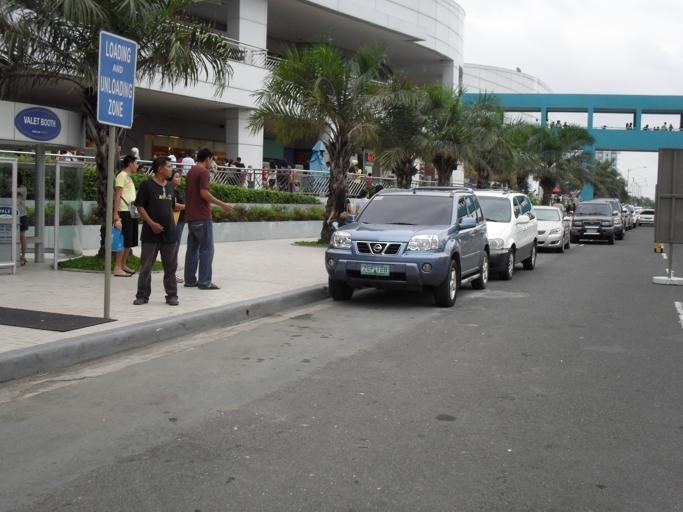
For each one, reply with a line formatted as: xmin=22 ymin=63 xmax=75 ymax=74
xmin=624 ymin=204 xmax=637 ymax=228
xmin=621 ymin=207 xmax=630 ymax=230
xmin=634 ymin=207 xmax=643 ymax=225
xmin=532 ymin=206 xmax=571 ymax=253
xmin=637 ymin=208 xmax=655 ymax=226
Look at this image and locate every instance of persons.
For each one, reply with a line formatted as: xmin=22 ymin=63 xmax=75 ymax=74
xmin=55 ymin=147 xmax=88 ymax=164
xmin=158 ymin=148 xmax=196 ymax=176
xmin=353 ymin=163 xmax=362 ymax=193
xmin=166 ymin=168 xmax=187 ymax=284
xmin=567 ymin=203 xmax=576 ymax=214
xmin=135 ymin=157 xmax=186 ymax=305
xmin=212 ymin=148 xmax=297 ymax=190
xmin=184 ymin=149 xmax=233 ymax=289
xmin=16 ymin=171 xmax=29 ymax=266
xmin=114 ymin=152 xmax=140 ymax=275
xmin=365 ymin=173 xmax=373 ymax=188
xmin=327 ymin=186 xmax=353 ymax=226
xmin=353 ymin=189 xmax=370 ymax=217
xmin=533 ymin=119 xmax=676 ymax=131
xmin=375 ymin=185 xmax=383 ymax=193
xmin=554 ymin=198 xmax=566 ymax=214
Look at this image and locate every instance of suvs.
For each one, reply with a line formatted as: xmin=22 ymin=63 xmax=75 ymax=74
xmin=570 ymin=202 xmax=616 ymax=245
xmin=591 ymin=198 xmax=625 ymax=240
xmin=473 ymin=190 xmax=539 ymax=281
xmin=325 ymin=186 xmax=491 ymax=309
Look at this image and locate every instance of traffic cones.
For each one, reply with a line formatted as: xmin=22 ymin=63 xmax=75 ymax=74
xmin=654 ymin=243 xmax=663 ymax=253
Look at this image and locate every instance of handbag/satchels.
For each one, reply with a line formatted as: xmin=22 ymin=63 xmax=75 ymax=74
xmin=120 ymin=194 xmax=140 ymax=218
xmin=112 ymin=222 xmax=124 ymax=253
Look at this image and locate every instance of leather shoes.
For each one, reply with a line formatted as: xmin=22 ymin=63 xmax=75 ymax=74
xmin=184 ymin=280 xmax=198 ymax=286
xmin=166 ymin=295 xmax=178 ymax=305
xmin=133 ymin=298 xmax=147 ymax=305
xmin=200 ymin=282 xmax=219 ymax=289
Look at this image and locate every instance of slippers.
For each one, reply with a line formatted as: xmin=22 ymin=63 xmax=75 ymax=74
xmin=124 ymin=266 xmax=135 ymax=274
xmin=115 ymin=272 xmax=131 ymax=277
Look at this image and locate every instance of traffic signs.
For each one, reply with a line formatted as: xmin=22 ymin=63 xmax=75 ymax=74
xmin=96 ymin=30 xmax=137 ymax=129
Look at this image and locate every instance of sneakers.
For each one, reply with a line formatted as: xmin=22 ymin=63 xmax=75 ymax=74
xmin=176 ymin=274 xmax=183 ymax=283
